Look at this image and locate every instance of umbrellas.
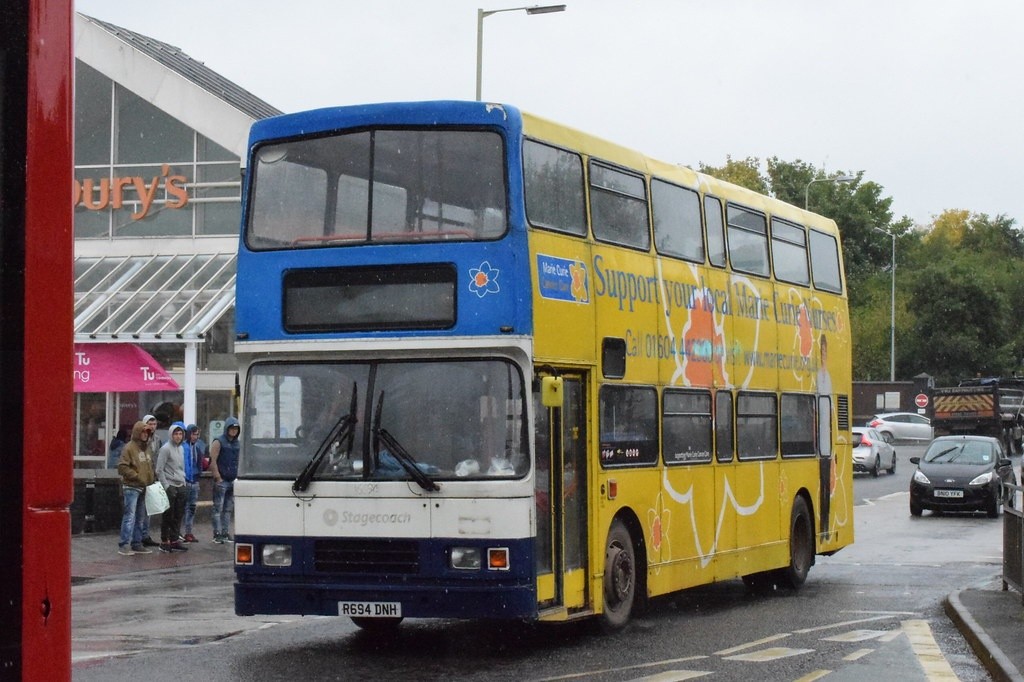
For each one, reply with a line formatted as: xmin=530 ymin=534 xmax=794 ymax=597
xmin=73 ymin=343 xmax=180 ymax=393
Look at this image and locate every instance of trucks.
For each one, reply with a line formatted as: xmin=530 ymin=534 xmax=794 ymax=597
xmin=929 ymin=380 xmax=1024 ymax=456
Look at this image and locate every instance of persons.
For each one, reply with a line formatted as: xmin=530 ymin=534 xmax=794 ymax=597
xmin=816 ymin=334 xmax=834 ymax=545
xmin=211 ymin=417 xmax=241 ymax=545
xmin=107 ymin=425 xmax=134 ymax=469
xmin=117 ymin=415 xmax=212 ymax=556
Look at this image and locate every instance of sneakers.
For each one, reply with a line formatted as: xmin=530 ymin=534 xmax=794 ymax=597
xmin=142 ymin=536 xmax=160 ymax=547
xmin=117 ymin=543 xmax=135 ymax=556
xmin=131 ymin=543 xmax=152 ymax=553
xmin=184 ymin=533 xmax=199 ymax=542
xmin=224 ymin=534 xmax=234 ymax=542
xmin=212 ymin=535 xmax=224 ymax=544
xmin=171 ymin=541 xmax=188 ymax=552
xmin=158 ymin=543 xmax=172 ymax=553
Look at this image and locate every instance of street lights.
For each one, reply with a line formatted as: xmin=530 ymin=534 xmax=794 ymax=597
xmin=875 ymin=227 xmax=897 ymax=381
xmin=474 ymin=3 xmax=567 ymax=108
xmin=804 ymin=176 xmax=857 ymax=212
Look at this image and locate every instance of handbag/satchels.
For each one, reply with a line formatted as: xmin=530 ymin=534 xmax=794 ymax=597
xmin=145 ymin=480 xmax=171 ymax=516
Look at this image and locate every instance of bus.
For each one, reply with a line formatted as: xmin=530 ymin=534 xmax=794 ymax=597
xmin=220 ymin=101 xmax=862 ymax=635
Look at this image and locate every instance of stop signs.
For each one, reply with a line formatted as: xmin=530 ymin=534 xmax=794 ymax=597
xmin=916 ymin=394 xmax=930 ymax=408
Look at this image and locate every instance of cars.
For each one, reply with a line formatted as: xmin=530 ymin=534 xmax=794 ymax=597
xmin=907 ymin=435 xmax=1017 ymax=518
xmin=849 ymin=426 xmax=898 ymax=472
xmin=865 ymin=413 xmax=934 ymax=444
